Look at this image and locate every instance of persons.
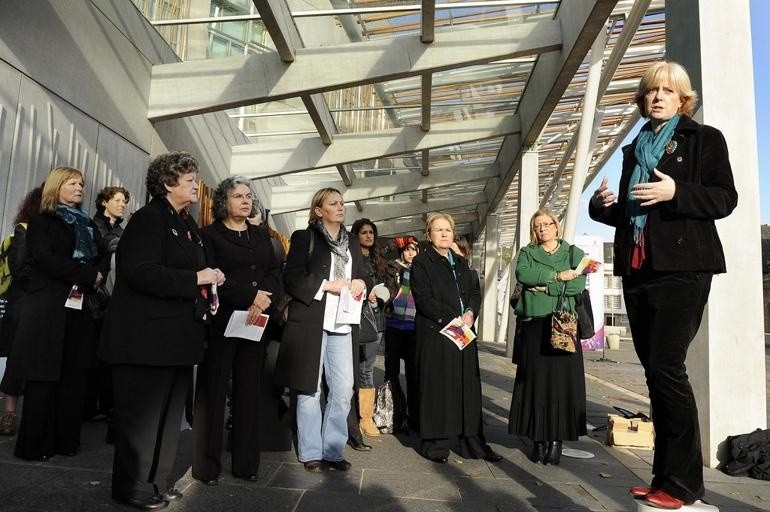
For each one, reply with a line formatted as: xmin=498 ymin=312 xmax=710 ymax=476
xmin=284 ymin=187 xmax=418 ymax=472
xmin=1 ymin=153 xmax=289 ymax=511
xmin=410 ymin=213 xmax=502 ymax=462
xmin=589 ymin=62 xmax=738 ymax=509
xmin=507 ymin=209 xmax=586 ymax=466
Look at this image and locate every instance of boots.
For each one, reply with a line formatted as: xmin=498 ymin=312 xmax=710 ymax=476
xmin=532 ymin=441 xmax=546 ymax=463
xmin=544 ymin=441 xmax=562 ymax=465
xmin=358 ymin=388 xmax=380 ymax=437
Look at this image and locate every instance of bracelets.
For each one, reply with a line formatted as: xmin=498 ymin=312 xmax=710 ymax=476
xmin=557 ymin=271 xmax=563 ymax=282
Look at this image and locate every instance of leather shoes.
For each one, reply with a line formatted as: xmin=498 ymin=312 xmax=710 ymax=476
xmin=630 ymin=487 xmax=653 ymax=498
xmin=113 ymin=496 xmax=168 ymax=511
xmin=435 ymin=458 xmax=449 ymax=463
xmin=644 ymin=489 xmax=694 ymax=509
xmin=487 ymin=456 xmax=502 ymax=462
xmin=202 ymin=479 xmax=219 ymax=485
xmin=322 ymin=459 xmax=352 ymax=471
xmin=305 ymin=461 xmax=324 ymax=473
xmin=348 ymin=440 xmax=372 ymax=451
xmin=244 ymin=474 xmax=259 ymax=481
xmin=158 ymin=490 xmax=183 ymax=501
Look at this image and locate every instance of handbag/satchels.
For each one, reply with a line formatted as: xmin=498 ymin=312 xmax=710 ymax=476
xmin=549 ymin=311 xmax=578 ymax=354
xmin=84 ymin=283 xmax=110 ymax=327
xmin=573 ymin=289 xmax=595 ymax=339
xmin=374 ymin=378 xmax=405 ymax=434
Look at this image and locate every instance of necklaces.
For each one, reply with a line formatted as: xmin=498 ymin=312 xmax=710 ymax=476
xmin=545 ymin=241 xmax=559 ymax=255
